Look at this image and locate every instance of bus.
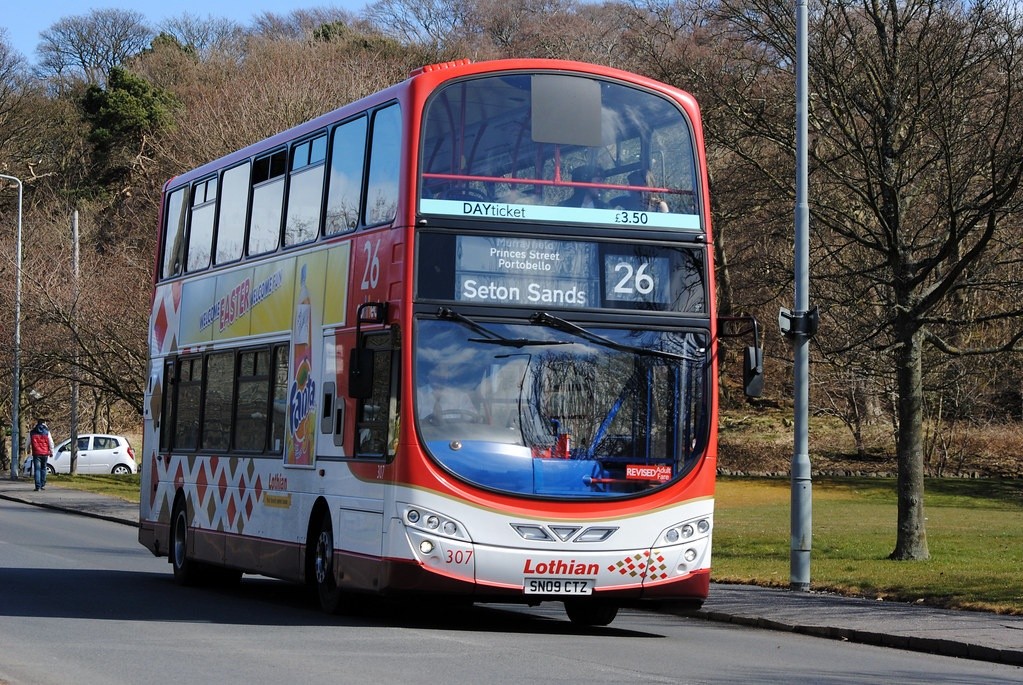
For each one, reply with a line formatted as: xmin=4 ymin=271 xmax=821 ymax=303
xmin=138 ymin=62 xmax=717 ymax=624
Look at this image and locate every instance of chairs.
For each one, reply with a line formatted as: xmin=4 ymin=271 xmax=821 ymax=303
xmin=443 ymin=188 xmax=490 ymax=202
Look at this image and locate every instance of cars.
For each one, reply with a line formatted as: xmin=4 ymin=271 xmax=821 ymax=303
xmin=22 ymin=433 xmax=139 ymax=475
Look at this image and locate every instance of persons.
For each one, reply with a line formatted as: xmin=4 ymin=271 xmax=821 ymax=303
xmin=415 ymin=354 xmax=484 ymax=427
xmin=555 ymin=161 xmax=617 ymax=211
xmin=611 ymin=169 xmax=671 ymax=215
xmin=67 ymin=440 xmax=88 ymax=451
xmin=27 ymin=418 xmax=55 ymax=491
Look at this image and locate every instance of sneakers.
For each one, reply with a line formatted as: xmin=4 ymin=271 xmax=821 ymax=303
xmin=34 ymin=486 xmax=39 ymax=491
xmin=41 ymin=486 xmax=45 ymax=490
